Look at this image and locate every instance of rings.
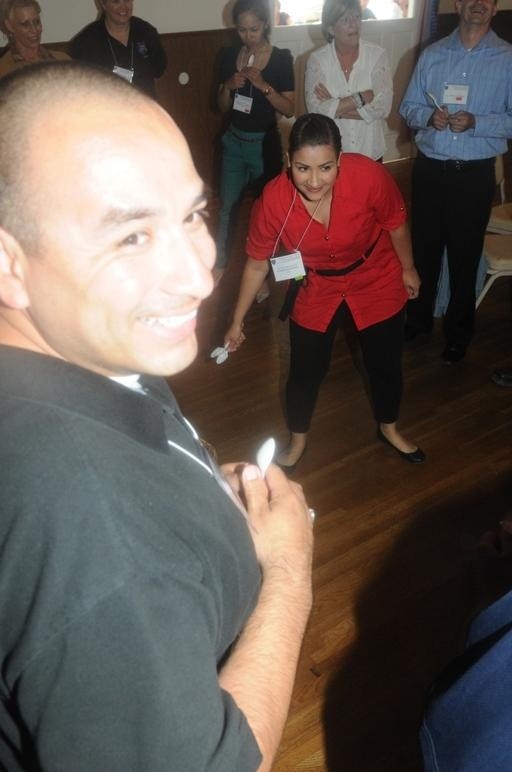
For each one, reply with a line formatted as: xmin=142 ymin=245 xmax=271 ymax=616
xmin=309 ymin=509 xmax=316 ymax=521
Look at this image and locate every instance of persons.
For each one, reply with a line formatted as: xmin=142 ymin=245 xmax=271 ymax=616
xmin=304 ymin=0 xmax=393 ymax=166
xmin=417 ymin=513 xmax=512 ymax=772
xmin=224 ymin=114 xmax=426 ymax=478
xmin=274 ymin=0 xmax=293 ymax=25
xmin=398 ymin=0 xmax=512 ymax=362
xmin=211 ymin=0 xmax=295 ymax=288
xmin=359 ymin=0 xmax=377 ymax=20
xmin=0 ymin=0 xmax=72 ymax=80
xmin=68 ymin=0 xmax=167 ymax=100
xmin=0 ymin=60 xmax=313 ymax=772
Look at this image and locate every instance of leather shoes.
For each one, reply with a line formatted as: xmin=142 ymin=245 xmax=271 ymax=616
xmin=254 ymin=292 xmax=270 ymax=304
xmin=377 ymin=423 xmax=426 ymax=462
xmin=403 ymin=322 xmax=467 ymax=364
xmin=273 ymin=438 xmax=307 ymax=476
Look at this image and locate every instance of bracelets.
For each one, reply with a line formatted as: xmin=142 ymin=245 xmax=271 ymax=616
xmin=353 ymin=92 xmax=365 ymax=108
xmin=263 ymin=85 xmax=271 ymax=96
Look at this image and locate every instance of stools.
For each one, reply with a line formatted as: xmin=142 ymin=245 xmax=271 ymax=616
xmin=474 ymin=234 xmax=512 ymax=310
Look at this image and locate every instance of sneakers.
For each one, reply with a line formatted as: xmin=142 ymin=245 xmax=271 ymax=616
xmin=489 ymin=366 xmax=512 ymax=387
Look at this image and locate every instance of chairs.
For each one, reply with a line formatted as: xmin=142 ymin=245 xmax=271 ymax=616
xmin=486 ymin=155 xmax=512 ymax=234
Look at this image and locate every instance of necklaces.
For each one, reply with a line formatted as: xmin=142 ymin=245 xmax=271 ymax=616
xmin=339 ymin=55 xmax=355 ymax=73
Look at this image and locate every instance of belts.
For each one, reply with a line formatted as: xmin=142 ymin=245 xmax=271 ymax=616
xmin=226 ymin=128 xmax=264 ymax=144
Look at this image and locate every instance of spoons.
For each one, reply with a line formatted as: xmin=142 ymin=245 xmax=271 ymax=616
xmin=425 ymin=92 xmax=444 ymax=113
xmin=247 ymin=54 xmax=255 ymax=67
xmin=210 ymin=341 xmax=230 ymax=359
xmin=256 ymin=436 xmax=277 ymax=477
xmin=216 ymin=351 xmax=228 ymax=365
